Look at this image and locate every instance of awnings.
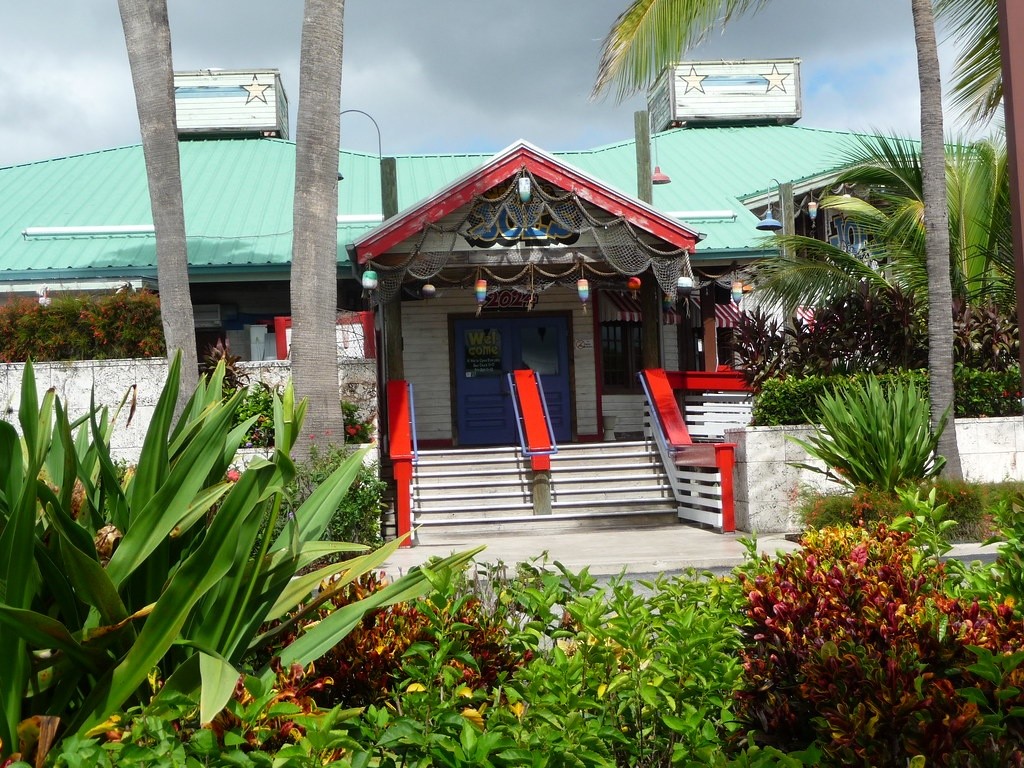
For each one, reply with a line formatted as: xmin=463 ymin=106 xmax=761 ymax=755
xmin=598 ymin=289 xmax=755 ymax=328
xmin=798 ymin=305 xmax=827 ymax=332
